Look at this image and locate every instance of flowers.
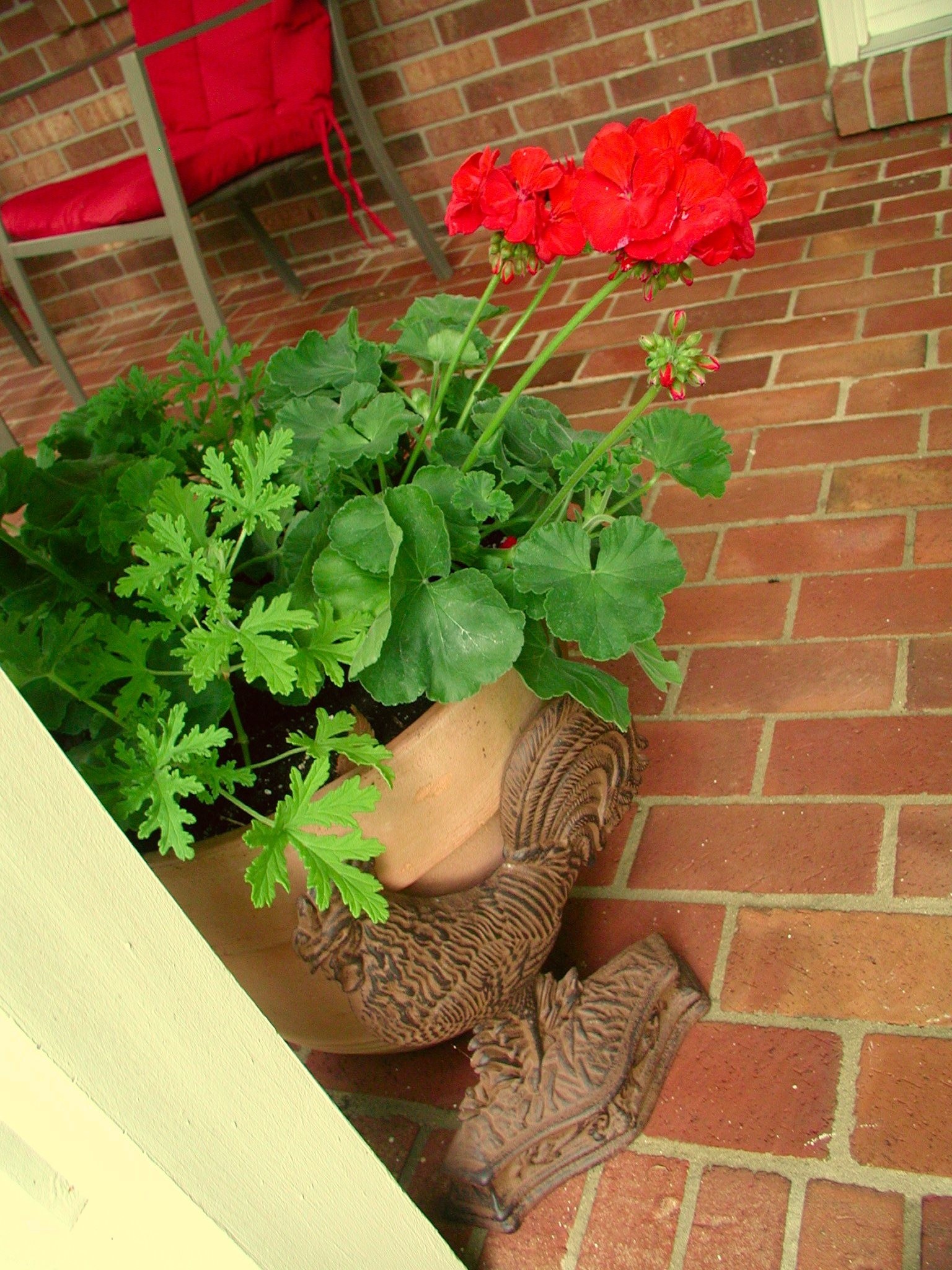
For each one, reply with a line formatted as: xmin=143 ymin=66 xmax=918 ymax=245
xmin=0 ymin=94 xmax=773 ymax=924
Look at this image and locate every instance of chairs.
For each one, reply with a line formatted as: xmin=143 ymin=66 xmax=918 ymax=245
xmin=0 ymin=0 xmax=451 ymax=412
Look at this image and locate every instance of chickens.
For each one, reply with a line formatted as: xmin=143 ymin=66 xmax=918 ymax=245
xmin=290 ymin=694 xmax=650 ymax=1054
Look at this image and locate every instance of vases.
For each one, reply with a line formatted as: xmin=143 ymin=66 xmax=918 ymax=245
xmin=136 ymin=631 xmax=721 ymax=1227
